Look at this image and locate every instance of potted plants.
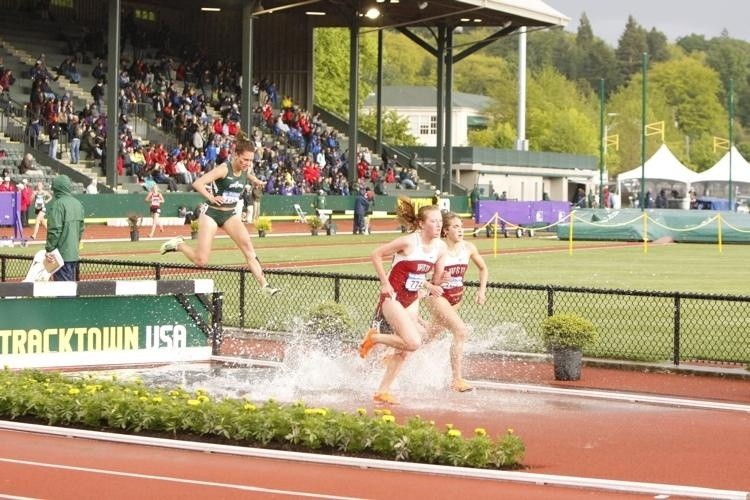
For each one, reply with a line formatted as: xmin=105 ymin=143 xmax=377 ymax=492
xmin=540 ymin=313 xmax=599 ymax=381
xmin=306 ymin=214 xmax=321 ymax=235
xmin=252 ymin=212 xmax=271 ymax=237
xmin=126 ymin=213 xmax=142 ymax=243
xmin=190 ymin=219 xmax=199 ymax=241
xmin=305 ymin=298 xmax=349 ymax=358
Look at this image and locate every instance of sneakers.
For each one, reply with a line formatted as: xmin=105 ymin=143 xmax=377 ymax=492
xmin=451 ymin=378 xmax=474 ymax=392
xmin=160 ymin=236 xmax=184 ymax=255
xmin=260 ymin=285 xmax=280 ymax=296
xmin=359 ymin=329 xmax=378 ymax=358
xmin=374 ymin=393 xmax=398 ymax=405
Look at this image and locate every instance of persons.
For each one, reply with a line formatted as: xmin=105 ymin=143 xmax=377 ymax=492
xmin=45 ymin=175 xmax=85 ymax=281
xmin=434 ymin=188 xmax=452 ymax=210
xmin=470 ymin=181 xmax=508 ymax=209
xmin=542 ymin=186 xmax=698 ymax=209
xmin=359 ymin=197 xmax=451 ymax=404
xmin=381 ymin=210 xmax=488 ymax=392
xmin=160 ymin=131 xmax=281 ymax=296
xmin=737 ymin=202 xmax=750 ymax=212
xmin=1 ymin=52 xmax=211 ymax=242
xmin=244 ymin=181 xmax=375 ymax=238
xmin=73 ymin=32 xmax=420 ymax=196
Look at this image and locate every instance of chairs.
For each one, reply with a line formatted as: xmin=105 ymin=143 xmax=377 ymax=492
xmin=293 ymin=203 xmax=307 ymax=223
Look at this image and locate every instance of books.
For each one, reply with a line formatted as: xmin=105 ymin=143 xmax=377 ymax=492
xmin=43 ymin=255 xmax=60 ymax=273
